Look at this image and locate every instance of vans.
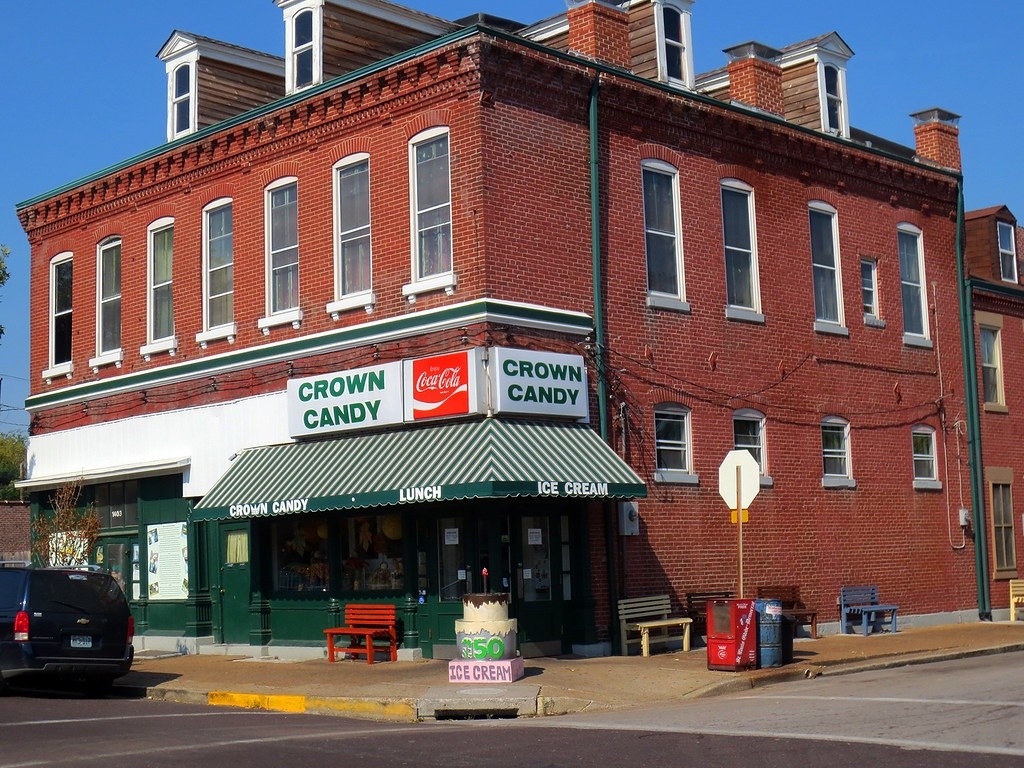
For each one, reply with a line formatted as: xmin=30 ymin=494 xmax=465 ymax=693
xmin=0 ymin=564 xmax=135 ymax=696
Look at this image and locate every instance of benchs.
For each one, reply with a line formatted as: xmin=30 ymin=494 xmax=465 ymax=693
xmin=1009 ymin=579 xmax=1024 ymax=621
xmin=758 ymin=585 xmax=819 ymax=639
xmin=685 ymin=591 xmax=734 ymax=648
xmin=840 ymin=586 xmax=899 ymax=637
xmin=617 ymin=594 xmax=693 ymax=658
xmin=323 ymin=604 xmax=397 ymax=664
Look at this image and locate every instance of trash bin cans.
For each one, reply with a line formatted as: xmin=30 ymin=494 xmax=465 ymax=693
xmin=782 ymin=612 xmax=797 ymax=664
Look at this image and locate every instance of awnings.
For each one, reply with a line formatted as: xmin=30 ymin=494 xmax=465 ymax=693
xmin=189 ymin=418 xmax=647 ymax=522
